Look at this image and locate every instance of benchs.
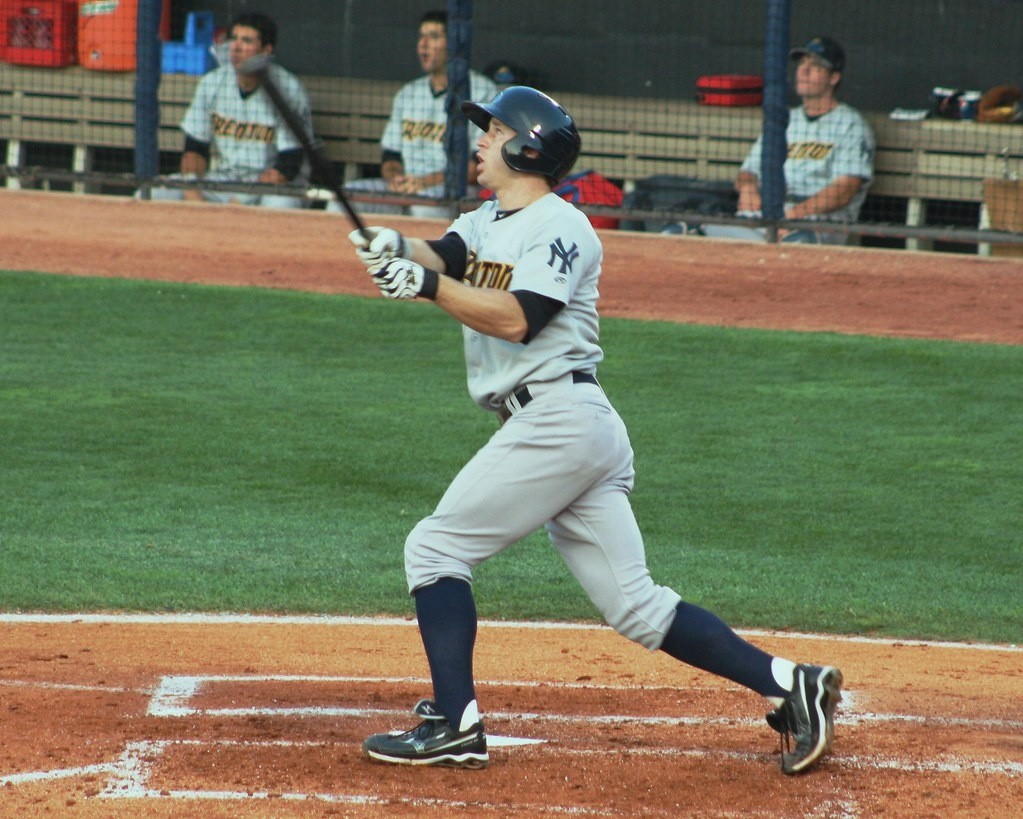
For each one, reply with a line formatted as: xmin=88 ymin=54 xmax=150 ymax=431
xmin=1 ymin=58 xmax=1023 ymax=258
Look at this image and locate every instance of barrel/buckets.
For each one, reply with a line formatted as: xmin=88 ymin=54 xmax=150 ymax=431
xmin=77 ymin=0 xmax=172 ymax=73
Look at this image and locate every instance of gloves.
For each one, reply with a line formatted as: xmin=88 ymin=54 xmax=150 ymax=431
xmin=349 ymin=225 xmax=406 ymax=268
xmin=367 ymin=256 xmax=431 ymax=299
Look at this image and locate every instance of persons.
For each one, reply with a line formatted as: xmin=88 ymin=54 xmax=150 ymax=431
xmin=378 ymin=12 xmax=506 ymax=225
xmin=696 ymin=36 xmax=878 ymax=250
xmin=131 ymin=10 xmax=313 ymax=208
xmin=349 ymin=84 xmax=841 ymax=774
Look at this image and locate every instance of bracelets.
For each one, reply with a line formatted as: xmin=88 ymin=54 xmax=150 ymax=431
xmin=418 ymin=265 xmax=438 ymax=300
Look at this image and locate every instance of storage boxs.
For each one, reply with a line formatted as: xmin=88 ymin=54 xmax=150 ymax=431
xmin=0 ymin=0 xmax=170 ymax=72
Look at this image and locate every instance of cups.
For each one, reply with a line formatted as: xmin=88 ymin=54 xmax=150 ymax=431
xmin=929 ymin=87 xmax=982 ymax=121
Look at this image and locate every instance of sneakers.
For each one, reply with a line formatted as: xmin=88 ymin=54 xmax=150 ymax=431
xmin=362 ymin=698 xmax=489 ymax=770
xmin=766 ymin=663 xmax=843 ymax=774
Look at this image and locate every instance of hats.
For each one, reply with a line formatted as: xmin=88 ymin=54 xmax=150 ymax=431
xmin=790 ymin=37 xmax=844 ymax=70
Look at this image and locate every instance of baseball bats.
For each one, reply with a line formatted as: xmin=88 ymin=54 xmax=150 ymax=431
xmin=239 ymin=51 xmax=375 ymax=240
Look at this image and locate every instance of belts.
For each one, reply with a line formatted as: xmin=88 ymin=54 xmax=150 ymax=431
xmin=496 ymin=371 xmax=600 ymax=425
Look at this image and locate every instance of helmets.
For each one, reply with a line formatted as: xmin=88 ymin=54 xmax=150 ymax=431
xmin=461 ymin=85 xmax=580 ymax=186
